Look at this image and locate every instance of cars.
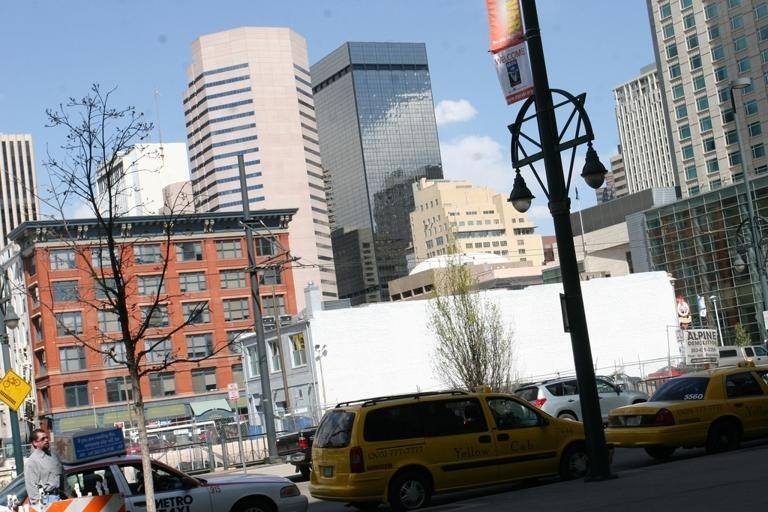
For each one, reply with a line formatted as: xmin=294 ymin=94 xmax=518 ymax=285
xmin=513 ymin=373 xmax=650 ymax=423
xmin=0 ymin=453 xmax=309 ymax=512
xmin=603 ymin=356 xmax=767 ymax=462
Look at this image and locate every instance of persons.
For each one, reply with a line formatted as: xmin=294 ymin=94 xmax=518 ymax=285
xmin=24 ymin=429 xmax=75 ymax=506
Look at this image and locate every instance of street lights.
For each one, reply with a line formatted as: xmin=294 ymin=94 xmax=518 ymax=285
xmin=733 ymin=215 xmax=768 ymax=343
xmin=505 ymin=88 xmax=612 ymax=483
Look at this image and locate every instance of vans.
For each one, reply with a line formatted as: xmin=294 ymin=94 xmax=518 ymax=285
xmin=307 ymin=385 xmax=616 ymax=511
xmin=719 ymin=344 xmax=767 ymax=365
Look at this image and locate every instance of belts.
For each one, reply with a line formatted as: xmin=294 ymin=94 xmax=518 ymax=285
xmin=44 ymin=490 xmax=58 ymax=495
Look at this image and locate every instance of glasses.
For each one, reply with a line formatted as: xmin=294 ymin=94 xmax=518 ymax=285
xmin=36 ymin=436 xmax=48 ymax=442
